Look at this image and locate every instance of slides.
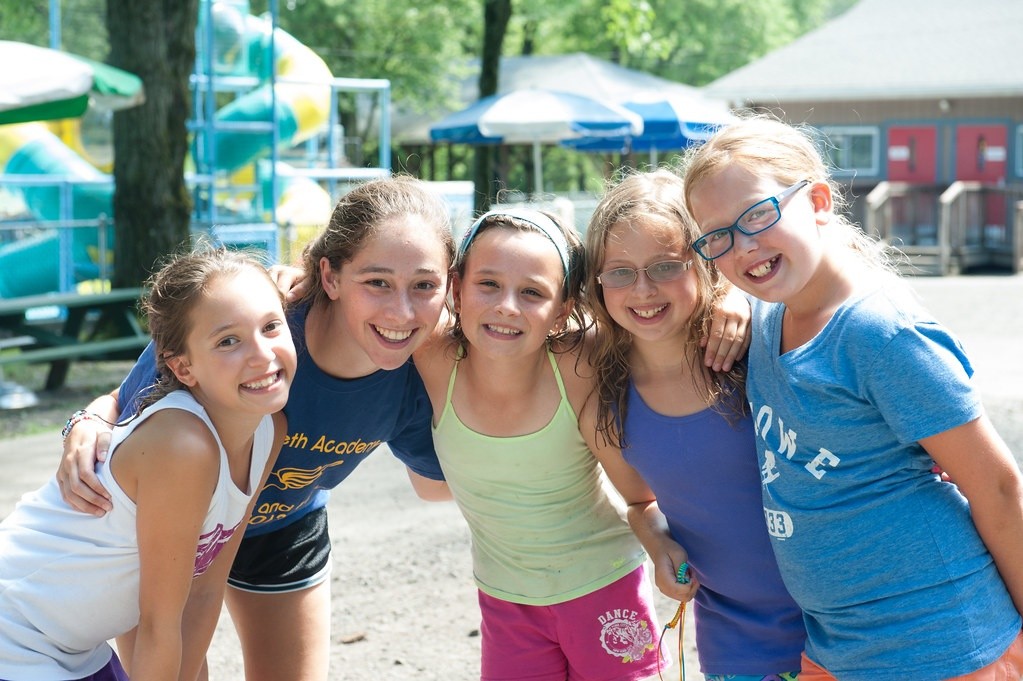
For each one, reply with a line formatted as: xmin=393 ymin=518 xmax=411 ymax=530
xmin=1 ymin=120 xmax=117 ymax=304
xmin=191 ymin=2 xmax=337 ymax=271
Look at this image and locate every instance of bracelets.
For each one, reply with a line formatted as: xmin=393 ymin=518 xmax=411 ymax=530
xmin=62 ymin=410 xmax=93 ymax=448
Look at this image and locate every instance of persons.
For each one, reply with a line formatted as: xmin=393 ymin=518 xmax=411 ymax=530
xmin=686 ymin=120 xmax=1023 ymax=681
xmin=56 ymin=176 xmax=455 ymax=681
xmin=0 ymin=247 xmax=300 ymax=681
xmin=268 ymin=204 xmax=752 ymax=681
xmin=578 ymin=170 xmax=807 ymax=681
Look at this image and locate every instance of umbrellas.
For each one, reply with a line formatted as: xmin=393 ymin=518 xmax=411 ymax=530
xmin=0 ymin=39 xmax=145 ymax=125
xmin=398 ymin=52 xmax=742 ymax=189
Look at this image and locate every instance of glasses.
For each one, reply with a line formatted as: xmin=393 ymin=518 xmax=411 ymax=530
xmin=691 ymin=179 xmax=807 ymax=262
xmin=595 ymin=258 xmax=694 ymax=289
